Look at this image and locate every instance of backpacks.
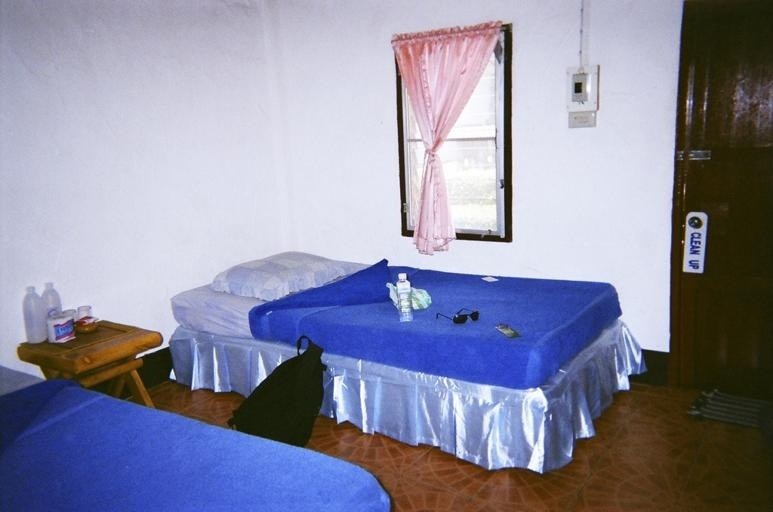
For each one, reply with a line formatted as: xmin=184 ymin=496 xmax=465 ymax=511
xmin=226 ymin=335 xmax=328 ymax=448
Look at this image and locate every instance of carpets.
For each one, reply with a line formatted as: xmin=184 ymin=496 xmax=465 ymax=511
xmin=687 ymin=386 xmax=772 ymax=430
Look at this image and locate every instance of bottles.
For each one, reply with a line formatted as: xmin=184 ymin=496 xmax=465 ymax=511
xmin=23 ymin=286 xmax=47 ymax=344
xmin=396 ymin=273 xmax=414 ymax=323
xmin=41 ymin=283 xmax=62 ymax=317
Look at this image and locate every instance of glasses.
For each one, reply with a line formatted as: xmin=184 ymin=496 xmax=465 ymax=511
xmin=436 ymin=308 xmax=480 ymax=324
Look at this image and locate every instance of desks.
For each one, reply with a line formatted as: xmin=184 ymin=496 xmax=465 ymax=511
xmin=16 ymin=322 xmax=163 ymax=408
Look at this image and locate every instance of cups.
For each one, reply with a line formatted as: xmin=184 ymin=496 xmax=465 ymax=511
xmin=63 ymin=309 xmax=78 ymax=323
xmin=77 ymin=306 xmax=92 ymax=320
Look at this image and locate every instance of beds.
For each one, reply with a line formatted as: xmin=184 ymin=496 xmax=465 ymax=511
xmin=0 ymin=367 xmax=391 ymax=512
xmin=171 ymin=261 xmax=617 ymax=473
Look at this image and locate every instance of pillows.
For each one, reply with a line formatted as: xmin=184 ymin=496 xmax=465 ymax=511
xmin=211 ymin=252 xmax=346 ymax=302
